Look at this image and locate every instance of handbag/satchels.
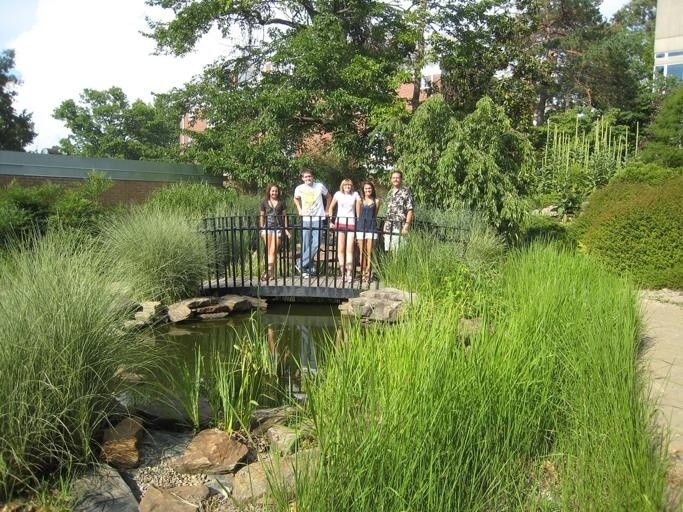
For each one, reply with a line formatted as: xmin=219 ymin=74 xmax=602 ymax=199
xmin=282 ymin=236 xmax=290 ymax=248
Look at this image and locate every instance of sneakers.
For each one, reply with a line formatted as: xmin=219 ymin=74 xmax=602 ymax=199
xmin=295 ymin=264 xmax=319 ymax=278
xmin=261 ymin=269 xmax=274 ymax=281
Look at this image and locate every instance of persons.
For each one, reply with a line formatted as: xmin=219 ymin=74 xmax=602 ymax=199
xmin=260 ymin=184 xmax=291 ymax=282
xmin=354 ymin=181 xmax=381 ymax=281
xmin=383 ymin=170 xmax=413 ymax=259
xmin=293 ymin=168 xmax=332 ymax=279
xmin=327 ymin=178 xmax=362 ymax=283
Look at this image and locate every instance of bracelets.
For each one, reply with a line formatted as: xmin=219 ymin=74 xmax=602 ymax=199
xmin=405 ymin=221 xmax=411 ymax=225
